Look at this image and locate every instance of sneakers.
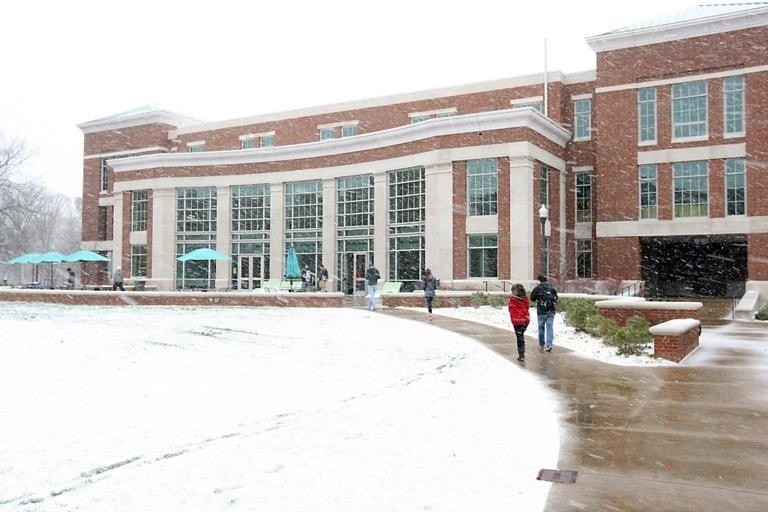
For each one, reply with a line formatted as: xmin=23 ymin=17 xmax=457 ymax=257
xmin=517 ymin=356 xmax=524 ymax=361
xmin=539 ymin=345 xmax=552 ymax=352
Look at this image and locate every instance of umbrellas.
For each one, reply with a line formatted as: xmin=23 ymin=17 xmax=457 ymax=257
xmin=6 ymin=250 xmax=111 ymax=289
xmin=176 ymin=247 xmax=233 ymax=290
xmin=282 ymin=243 xmax=301 ymax=290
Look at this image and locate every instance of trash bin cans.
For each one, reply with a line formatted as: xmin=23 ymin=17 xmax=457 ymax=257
xmin=134 ymin=281 xmax=146 ymax=291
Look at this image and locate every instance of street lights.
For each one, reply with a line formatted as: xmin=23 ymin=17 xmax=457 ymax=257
xmin=539 ymin=205 xmax=549 ymax=276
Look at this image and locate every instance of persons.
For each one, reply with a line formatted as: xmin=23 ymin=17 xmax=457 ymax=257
xmin=111 ymin=266 xmax=125 ymax=291
xmin=365 ymin=260 xmax=380 ymax=311
xmin=530 ymin=275 xmax=558 ymax=351
xmin=301 ymin=263 xmax=328 ymax=292
xmin=422 ymin=269 xmax=437 ymax=314
xmin=508 ymin=284 xmax=530 ymax=361
